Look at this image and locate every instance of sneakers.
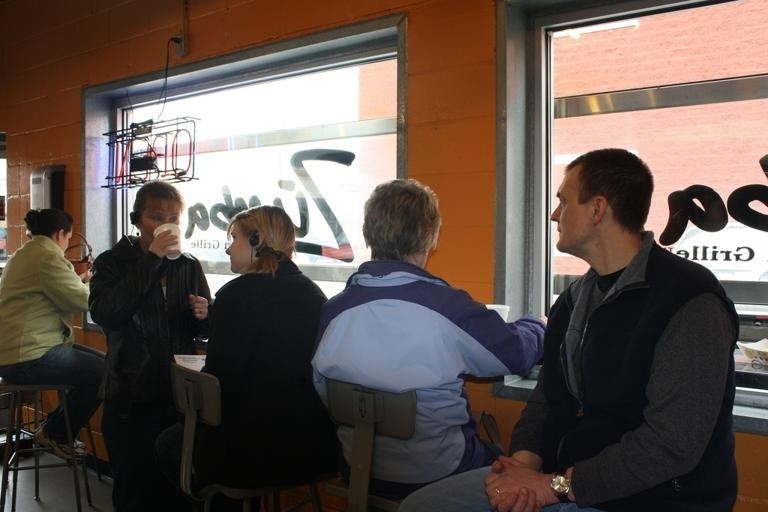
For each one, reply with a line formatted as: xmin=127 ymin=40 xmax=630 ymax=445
xmin=33 ymin=420 xmax=88 ymax=461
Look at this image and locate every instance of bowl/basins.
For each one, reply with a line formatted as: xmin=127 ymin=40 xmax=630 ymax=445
xmin=736 ymin=337 xmax=768 ymax=367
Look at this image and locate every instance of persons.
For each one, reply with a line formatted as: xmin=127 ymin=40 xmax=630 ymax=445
xmin=308 ymin=179 xmax=548 ymax=508
xmin=86 ymin=182 xmax=213 ymax=512
xmin=149 ymin=206 xmax=347 ymax=511
xmin=398 ymin=149 xmax=743 ymax=512
xmin=0 ymin=207 xmax=110 ymax=462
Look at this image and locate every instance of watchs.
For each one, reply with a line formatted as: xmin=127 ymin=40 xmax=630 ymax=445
xmin=549 ymin=466 xmax=574 ymax=503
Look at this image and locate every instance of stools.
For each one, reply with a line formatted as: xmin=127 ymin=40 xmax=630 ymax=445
xmin=0 ymin=369 xmax=103 ymax=510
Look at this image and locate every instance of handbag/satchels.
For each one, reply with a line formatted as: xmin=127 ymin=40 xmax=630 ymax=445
xmin=68 ymin=258 xmax=92 ymax=275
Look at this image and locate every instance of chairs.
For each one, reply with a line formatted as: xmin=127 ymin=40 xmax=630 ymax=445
xmin=169 ymin=362 xmax=337 ymax=510
xmin=328 ymin=378 xmax=416 ymax=510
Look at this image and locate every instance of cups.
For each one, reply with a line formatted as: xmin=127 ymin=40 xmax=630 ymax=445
xmin=152 ymin=222 xmax=183 ymax=261
xmin=485 ymin=303 xmax=511 ymax=323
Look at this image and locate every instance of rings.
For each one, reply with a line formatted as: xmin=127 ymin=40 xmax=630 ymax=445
xmin=496 ymin=485 xmax=504 ymax=495
xmin=199 ymin=308 xmax=204 ymax=314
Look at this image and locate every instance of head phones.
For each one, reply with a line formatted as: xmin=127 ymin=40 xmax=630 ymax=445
xmin=249 ymin=232 xmax=259 ymax=246
xmin=130 ymin=212 xmax=139 ymax=224
